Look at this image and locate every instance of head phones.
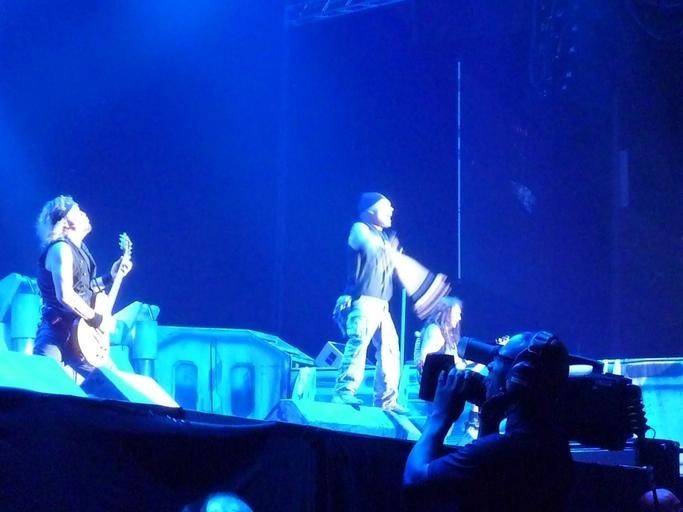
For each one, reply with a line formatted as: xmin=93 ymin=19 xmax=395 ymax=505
xmin=501 ymin=330 xmax=556 ymax=401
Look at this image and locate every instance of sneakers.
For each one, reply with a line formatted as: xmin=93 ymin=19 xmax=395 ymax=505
xmin=391 ymin=404 xmax=409 ymax=414
xmin=331 ymin=394 xmax=364 ymax=406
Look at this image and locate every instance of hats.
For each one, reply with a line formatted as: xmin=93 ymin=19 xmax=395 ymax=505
xmin=358 ymin=192 xmax=384 ymax=215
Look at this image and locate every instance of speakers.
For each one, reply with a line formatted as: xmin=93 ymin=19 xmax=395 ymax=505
xmin=260 ymin=397 xmax=399 ymax=437
xmin=0 ymin=350 xmax=90 ymax=400
xmin=82 ymin=366 xmax=181 ymax=407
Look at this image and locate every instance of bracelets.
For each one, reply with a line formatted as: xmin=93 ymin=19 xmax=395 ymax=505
xmin=100 ymin=272 xmax=113 ymax=287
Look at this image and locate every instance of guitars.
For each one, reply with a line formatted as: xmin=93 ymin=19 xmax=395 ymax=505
xmin=73 ymin=232 xmax=132 ymax=370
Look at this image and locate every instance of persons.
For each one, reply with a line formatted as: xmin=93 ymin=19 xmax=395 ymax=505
xmin=413 ymin=296 xmax=490 ymax=440
xmin=182 ymin=490 xmax=253 ymax=512
xmin=329 ymin=192 xmax=410 ymax=414
xmin=638 ymin=488 xmax=681 ymax=512
xmin=402 ymin=331 xmax=575 ymax=512
xmin=32 ymin=194 xmax=133 ymax=381
